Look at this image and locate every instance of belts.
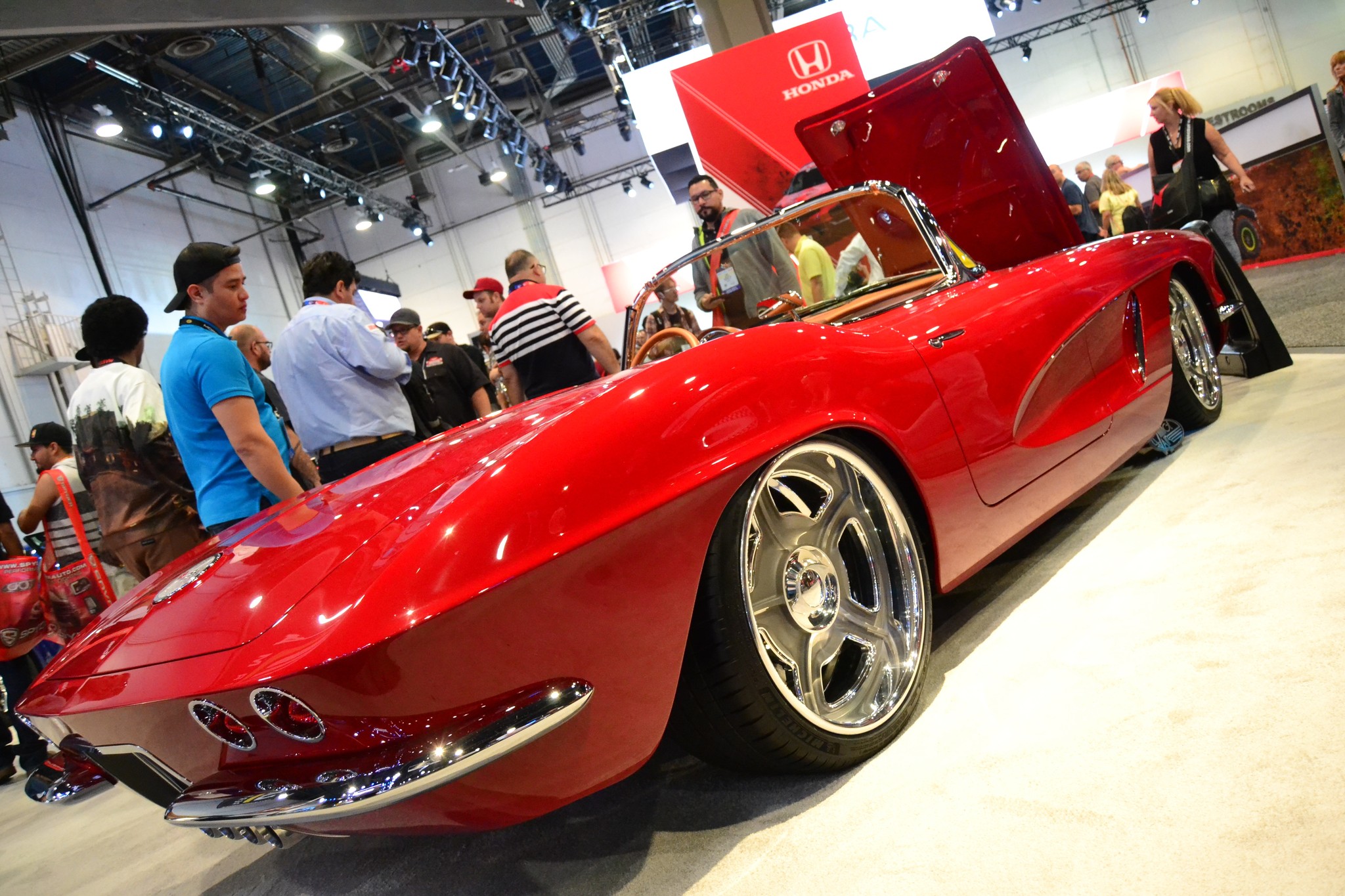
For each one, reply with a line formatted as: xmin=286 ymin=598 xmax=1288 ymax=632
xmin=318 ymin=432 xmax=402 ymax=455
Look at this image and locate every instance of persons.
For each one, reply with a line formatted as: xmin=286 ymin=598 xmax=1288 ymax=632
xmin=835 ymin=232 xmax=887 ymax=298
xmin=1075 ymin=161 xmax=1112 ymax=238
xmin=688 ymin=174 xmax=802 ymax=331
xmin=1106 ymin=155 xmax=1144 ymax=174
xmin=66 ymin=296 xmax=212 ymax=579
xmin=272 ymin=251 xmax=422 ymax=486
xmin=1326 ymin=50 xmax=1345 ymax=164
xmin=228 ymin=326 xmax=324 ymax=491
xmin=0 ymin=488 xmax=49 ymax=784
xmin=488 ymin=250 xmax=623 ymax=407
xmin=1048 ymin=164 xmax=1103 ymax=243
xmin=464 ymin=278 xmax=509 ymax=412
xmin=423 ymin=321 xmax=508 ymax=411
xmin=645 ymin=276 xmax=703 ymax=362
xmin=158 ymin=242 xmax=306 ymax=535
xmin=1099 ymin=169 xmax=1145 ymax=238
xmin=1147 ymin=87 xmax=1257 ymax=269
xmin=16 ymin=422 xmax=141 ymax=601
xmin=387 ymin=309 xmax=494 ymax=426
xmin=777 ymin=222 xmax=836 ymax=307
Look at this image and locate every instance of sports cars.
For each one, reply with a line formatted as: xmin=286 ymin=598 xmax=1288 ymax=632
xmin=13 ymin=30 xmax=1251 ymax=852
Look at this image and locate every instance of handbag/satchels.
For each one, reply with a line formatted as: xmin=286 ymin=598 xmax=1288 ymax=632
xmin=39 ymin=541 xmax=117 ymax=647
xmin=1152 ymin=159 xmax=1202 ymax=229
xmin=1 ymin=556 xmax=48 ymax=662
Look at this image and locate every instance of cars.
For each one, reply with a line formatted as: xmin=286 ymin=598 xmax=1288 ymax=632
xmin=774 ymin=160 xmax=855 ymax=248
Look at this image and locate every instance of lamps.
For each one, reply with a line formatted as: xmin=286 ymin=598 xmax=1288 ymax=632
xmin=346 ymin=187 xmax=363 ymax=206
xmin=365 ymin=206 xmax=383 ymax=222
xmin=405 ymin=217 xmax=422 ymax=236
xmin=421 ymin=227 xmax=433 ymax=247
xmin=615 ymin=90 xmax=627 ymax=112
xmin=1136 ymin=4 xmax=1149 ymax=24
xmin=621 ymin=86 xmax=629 ymax=105
xmin=688 ymin=7 xmax=701 ymax=25
xmin=306 ymin=187 xmax=326 ymax=200
xmin=318 ymin=29 xmax=344 ymax=52
xmin=618 ymin=121 xmax=631 ymax=142
xmin=986 ymin=0 xmax=1003 ymax=18
xmin=96 ymin=115 xmax=123 ymax=137
xmin=1032 ymin=0 xmax=1041 ymax=5
xmin=287 ymin=172 xmax=310 ymax=185
xmin=622 ymin=181 xmax=636 ymax=197
xmin=403 ymin=28 xmax=575 ymax=199
xmin=256 ymin=169 xmax=276 ymax=194
xmin=173 ymin=124 xmax=193 ymax=139
xmin=1014 ymin=0 xmax=1023 ymax=11
xmin=603 ymin=44 xmax=615 ymax=65
xmin=639 ymin=174 xmax=654 ymax=189
xmin=571 ymin=136 xmax=585 ymax=156
xmin=580 ymin=0 xmax=600 ymax=30
xmin=1000 ymin=0 xmax=1016 ymax=11
xmin=1020 ymin=42 xmax=1032 ymax=62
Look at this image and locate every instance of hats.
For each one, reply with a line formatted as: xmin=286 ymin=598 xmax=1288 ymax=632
xmin=463 ymin=278 xmax=503 ymax=299
xmin=164 ymin=243 xmax=241 ymax=313
xmin=75 ymin=295 xmax=148 ymax=361
xmin=16 ymin=422 xmax=71 ymax=448
xmin=385 ymin=308 xmax=420 ymax=330
xmin=424 ymin=322 xmax=450 ymax=339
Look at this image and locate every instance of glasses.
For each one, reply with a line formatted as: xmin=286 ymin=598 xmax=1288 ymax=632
xmin=689 ymin=189 xmax=717 ymax=204
xmin=391 ymin=326 xmax=415 ymax=337
xmin=1076 ymin=169 xmax=1086 ymax=174
xmin=531 ymin=263 xmax=547 ymax=274
xmin=258 ymin=342 xmax=273 ymax=350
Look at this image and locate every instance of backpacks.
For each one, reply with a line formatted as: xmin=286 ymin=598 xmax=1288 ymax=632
xmin=1121 ymin=206 xmax=1147 ymax=233
